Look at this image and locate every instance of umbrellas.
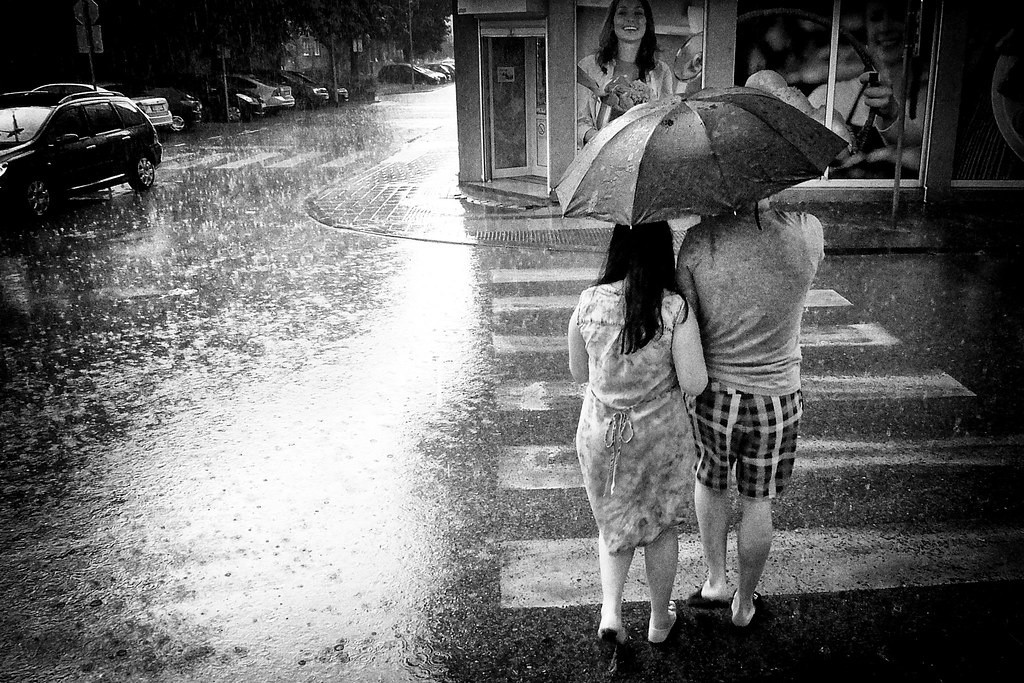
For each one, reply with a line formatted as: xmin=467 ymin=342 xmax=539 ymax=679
xmin=550 ymin=87 xmax=848 ymax=229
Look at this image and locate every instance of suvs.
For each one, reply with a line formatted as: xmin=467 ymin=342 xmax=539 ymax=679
xmin=0 ymin=82 xmax=164 ymax=219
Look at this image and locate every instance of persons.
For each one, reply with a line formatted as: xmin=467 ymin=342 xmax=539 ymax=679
xmin=573 ymin=0 xmax=926 ymax=181
xmin=569 ymin=218 xmax=708 ymax=646
xmin=678 ymin=195 xmax=824 ymax=632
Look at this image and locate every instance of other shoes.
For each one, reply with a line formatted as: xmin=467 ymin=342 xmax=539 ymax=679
xmin=731 ymin=589 xmax=755 ymax=625
xmin=598 ymin=612 xmax=624 ymax=644
xmin=701 ymin=578 xmax=724 ymax=597
xmin=648 ymin=601 xmax=678 ymax=642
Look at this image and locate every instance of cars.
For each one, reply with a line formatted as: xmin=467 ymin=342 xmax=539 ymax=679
xmin=378 ymin=61 xmax=454 ymax=85
xmin=203 ymin=70 xmax=349 ymax=121
xmin=101 ymin=82 xmax=202 ymax=133
